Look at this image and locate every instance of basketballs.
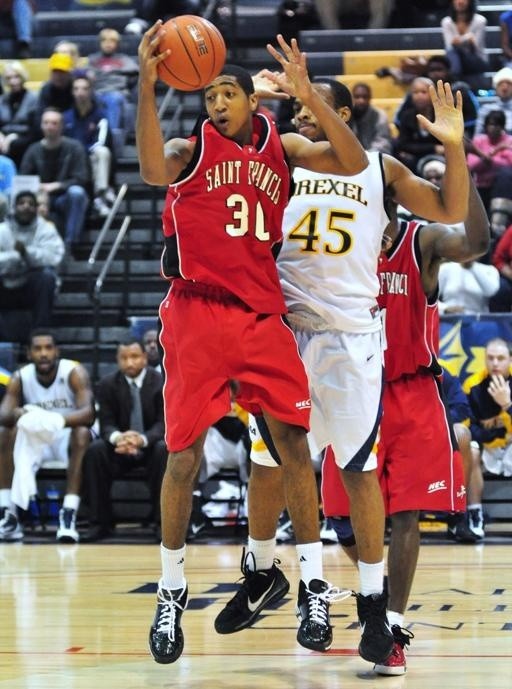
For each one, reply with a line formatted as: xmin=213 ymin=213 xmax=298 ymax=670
xmin=154 ymin=15 xmax=224 ymax=91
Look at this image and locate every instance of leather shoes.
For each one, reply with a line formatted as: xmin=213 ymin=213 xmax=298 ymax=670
xmin=79 ymin=526 xmax=113 ymax=542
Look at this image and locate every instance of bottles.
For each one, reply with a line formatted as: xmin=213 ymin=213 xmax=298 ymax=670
xmin=47 ymin=484 xmax=60 ymax=531
xmin=29 ymin=486 xmax=44 ymax=531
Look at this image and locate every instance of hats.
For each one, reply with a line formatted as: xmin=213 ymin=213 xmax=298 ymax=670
xmin=48 ymin=51 xmax=75 ymax=74
xmin=492 ymin=67 xmax=512 ymax=89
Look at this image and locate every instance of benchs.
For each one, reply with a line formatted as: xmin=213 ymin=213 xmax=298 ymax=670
xmin=0 ymin=0 xmax=511 ymax=543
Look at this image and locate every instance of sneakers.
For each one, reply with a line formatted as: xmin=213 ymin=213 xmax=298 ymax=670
xmin=215 ymin=562 xmax=291 ymax=634
xmin=275 ymin=516 xmax=340 ymax=544
xmin=93 ymin=197 xmax=109 ymax=216
xmin=56 ymin=507 xmax=80 ymax=542
xmin=186 ymin=511 xmax=216 ymax=541
xmin=147 ymin=576 xmax=189 ymax=665
xmin=0 ymin=509 xmax=26 ymax=541
xmin=351 ymin=590 xmax=395 ymax=664
xmin=295 ymin=577 xmax=335 ymax=653
xmin=373 ymin=625 xmax=411 ymax=676
xmin=446 ymin=504 xmax=486 ymax=543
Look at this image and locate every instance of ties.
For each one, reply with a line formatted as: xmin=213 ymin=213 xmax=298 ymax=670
xmin=130 ymin=384 xmax=145 ymax=437
